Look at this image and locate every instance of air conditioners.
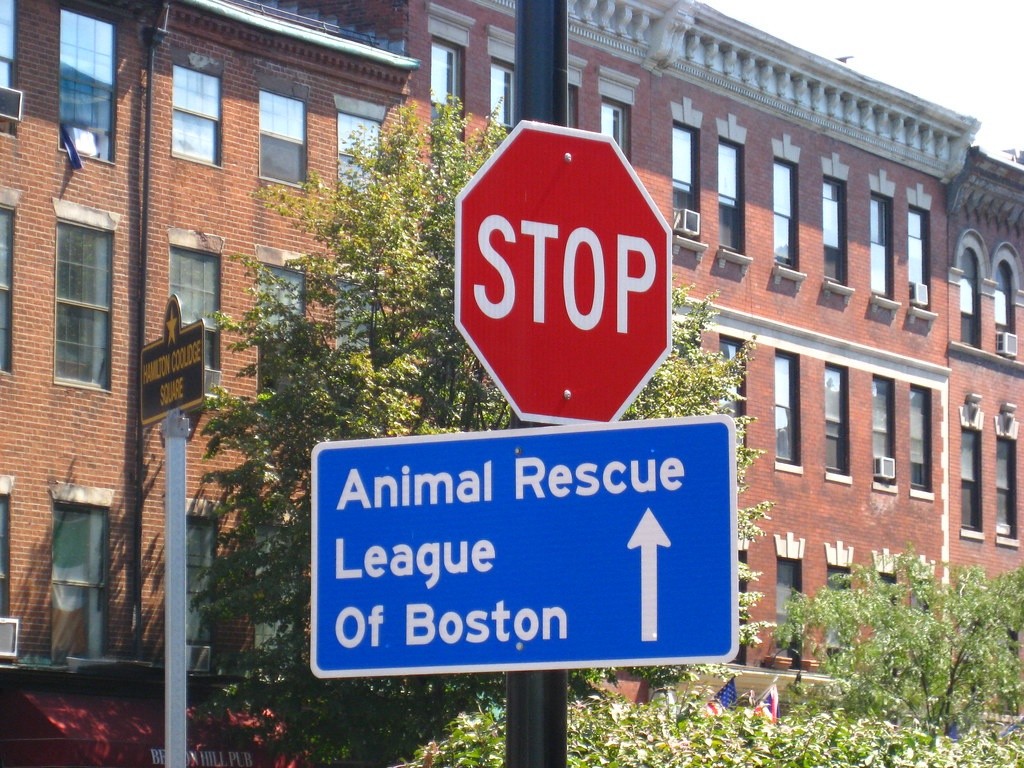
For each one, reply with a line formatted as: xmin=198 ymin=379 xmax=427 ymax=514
xmin=0 ymin=86 xmax=23 ymax=121
xmin=205 ymin=369 xmax=222 ymax=399
xmin=909 ymin=283 xmax=928 ymax=305
xmin=996 ymin=332 xmax=1018 ymax=357
xmin=0 ymin=618 xmax=19 ymax=659
xmin=186 ymin=645 xmax=210 ymax=672
xmin=673 ymin=208 xmax=700 ymax=235
xmin=872 ymin=456 xmax=896 ymax=480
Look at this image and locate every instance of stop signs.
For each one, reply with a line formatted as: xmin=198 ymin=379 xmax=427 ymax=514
xmin=455 ymin=120 xmax=673 ymax=424
xmin=312 ymin=415 xmax=739 ymax=678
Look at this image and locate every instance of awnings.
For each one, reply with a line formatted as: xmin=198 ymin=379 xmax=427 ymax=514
xmin=0 ymin=688 xmax=320 ymax=768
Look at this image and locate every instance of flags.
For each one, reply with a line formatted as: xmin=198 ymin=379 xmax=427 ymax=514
xmin=698 ymin=677 xmax=737 ymax=718
xmin=754 ymin=683 xmax=781 ymax=724
